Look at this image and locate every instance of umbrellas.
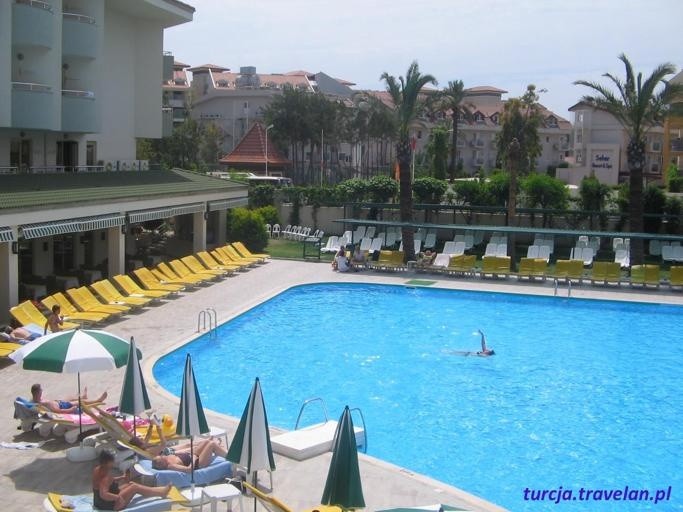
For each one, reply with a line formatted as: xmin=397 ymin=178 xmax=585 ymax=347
xmin=117 ymin=335 xmax=153 ymax=439
xmin=226 ymin=379 xmax=275 ymax=484
xmin=7 ymin=329 xmax=141 ymax=437
xmin=320 ymin=406 xmax=366 ymax=512
xmin=174 ymin=354 xmax=211 ymax=443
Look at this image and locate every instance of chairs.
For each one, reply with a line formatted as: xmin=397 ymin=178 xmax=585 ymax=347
xmin=265 ymin=223 xmax=325 ymax=247
xmin=322 ymin=223 xmax=682 ymax=291
xmin=1 ymin=239 xmax=271 ymax=356
xmin=12 ymin=395 xmax=234 ymax=512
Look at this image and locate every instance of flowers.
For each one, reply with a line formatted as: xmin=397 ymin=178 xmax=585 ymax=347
xmin=200 ymin=483 xmax=244 ymax=512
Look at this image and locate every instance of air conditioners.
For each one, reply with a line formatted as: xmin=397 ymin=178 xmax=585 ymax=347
xmin=97 ymin=160 xmax=150 ymax=173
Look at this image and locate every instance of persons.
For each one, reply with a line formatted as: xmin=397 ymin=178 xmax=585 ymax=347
xmin=92 ymin=449 xmax=172 ymax=512
xmin=446 ymin=329 xmax=496 ymax=358
xmin=129 ymin=414 xmax=230 ymax=474
xmin=30 ymin=384 xmax=108 ymax=413
xmin=331 ymin=245 xmax=357 ymax=272
xmin=0 ymin=305 xmax=65 ymax=342
xmin=420 ymin=248 xmax=436 ymax=271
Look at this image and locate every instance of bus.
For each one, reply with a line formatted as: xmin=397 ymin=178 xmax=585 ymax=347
xmin=203 ymin=171 xmax=296 ymax=189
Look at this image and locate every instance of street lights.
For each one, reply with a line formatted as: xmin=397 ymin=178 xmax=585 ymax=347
xmin=264 ymin=123 xmax=276 ymax=176
